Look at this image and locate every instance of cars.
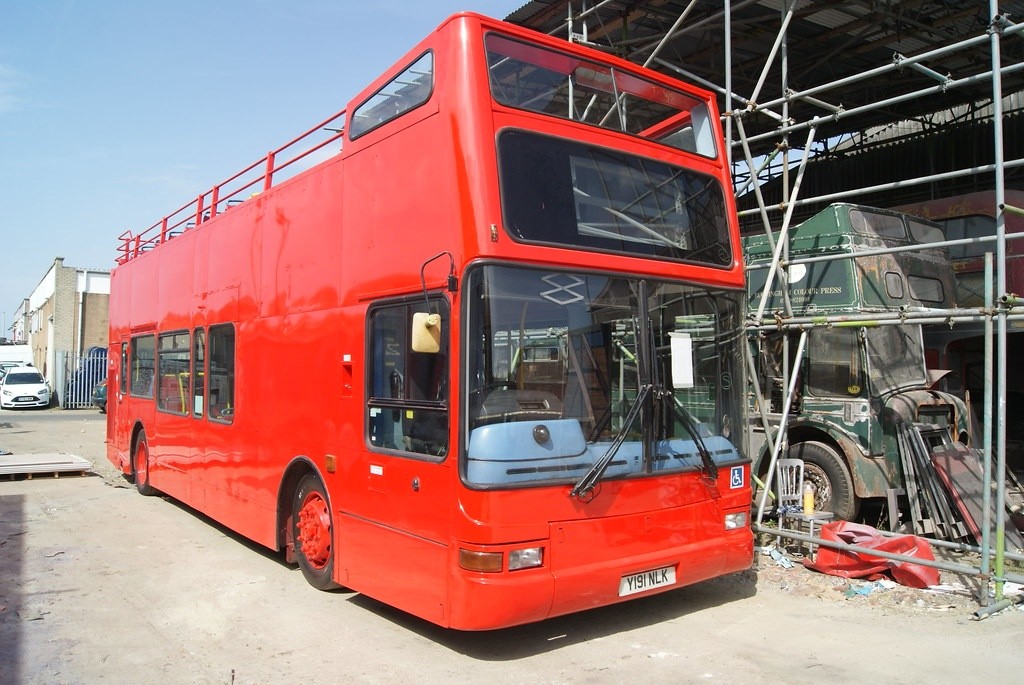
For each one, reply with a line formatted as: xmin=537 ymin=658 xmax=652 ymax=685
xmin=0 ymin=359 xmax=51 ymax=410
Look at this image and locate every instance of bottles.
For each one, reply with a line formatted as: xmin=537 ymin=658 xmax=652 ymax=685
xmin=804 ymin=484 xmax=814 ymax=514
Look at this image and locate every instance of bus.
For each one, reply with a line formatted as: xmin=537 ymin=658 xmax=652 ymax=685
xmin=742 ymin=202 xmax=1002 ymax=551
xmin=106 ymin=11 xmax=755 ymax=632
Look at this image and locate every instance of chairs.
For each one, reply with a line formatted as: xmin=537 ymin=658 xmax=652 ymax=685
xmin=131 ymin=358 xmax=234 ymax=423
xmin=776 ymin=458 xmax=834 ymax=564
xmin=119 ymin=199 xmax=244 ymax=266
xmin=402 ymin=353 xmax=449 ymax=456
